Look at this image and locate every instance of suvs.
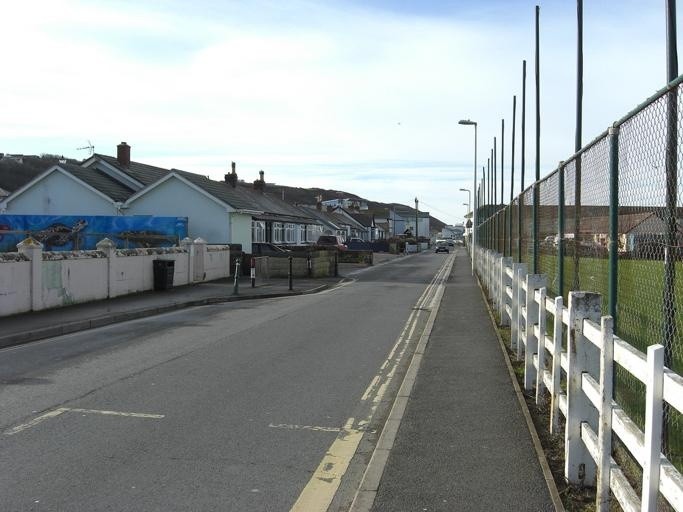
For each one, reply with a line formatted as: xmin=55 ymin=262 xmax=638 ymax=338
xmin=316 ymin=235 xmax=348 ymax=251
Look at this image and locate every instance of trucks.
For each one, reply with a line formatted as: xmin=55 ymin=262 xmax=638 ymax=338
xmin=395 ymin=232 xmax=412 ymax=252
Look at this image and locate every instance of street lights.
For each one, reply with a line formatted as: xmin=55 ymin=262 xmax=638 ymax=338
xmin=456 ymin=119 xmax=478 ymax=278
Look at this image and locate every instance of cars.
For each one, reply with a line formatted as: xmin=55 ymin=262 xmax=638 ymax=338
xmin=447 ymin=239 xmax=453 ymax=246
xmin=342 ymin=237 xmax=372 ymax=250
xmin=434 ymin=240 xmax=449 ymax=254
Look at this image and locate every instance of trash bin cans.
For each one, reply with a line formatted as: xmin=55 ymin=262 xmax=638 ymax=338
xmin=153 ymin=259 xmax=175 ymax=293
